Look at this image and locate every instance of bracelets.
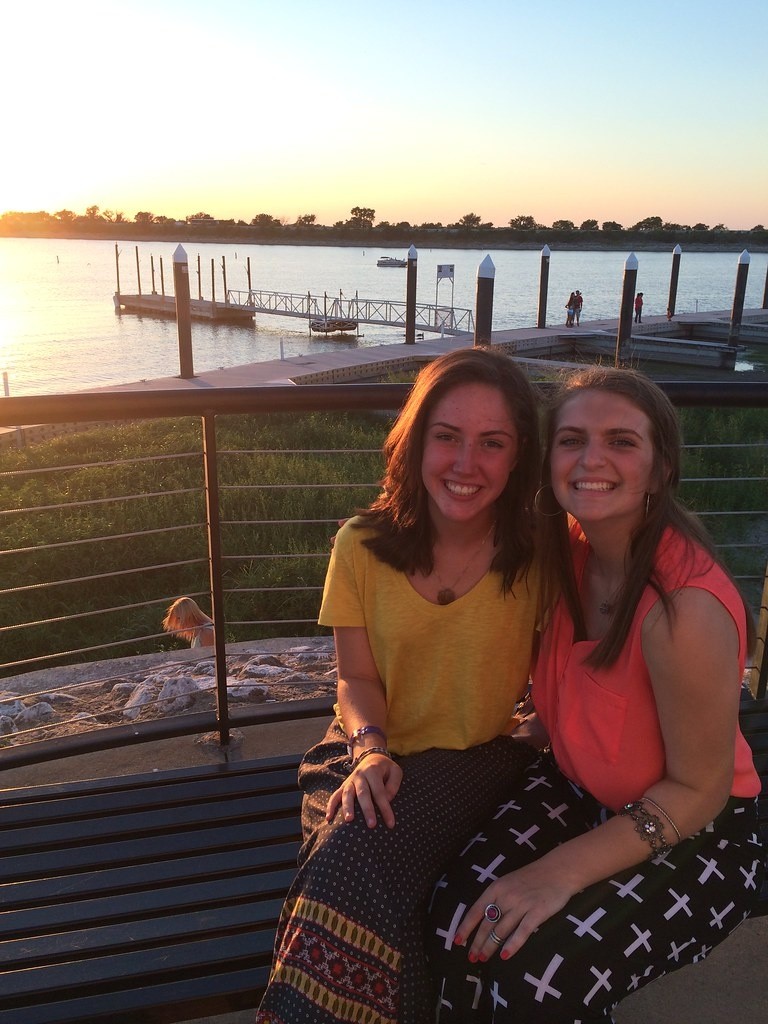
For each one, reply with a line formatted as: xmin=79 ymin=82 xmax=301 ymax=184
xmin=345 ymin=725 xmax=392 ymax=774
xmin=618 ymin=796 xmax=682 ymax=860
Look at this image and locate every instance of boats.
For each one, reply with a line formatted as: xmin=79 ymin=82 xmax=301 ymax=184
xmin=376 ymin=255 xmax=407 ymax=267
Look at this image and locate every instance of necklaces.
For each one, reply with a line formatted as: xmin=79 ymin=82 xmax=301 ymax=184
xmin=432 ymin=516 xmax=498 ymax=605
xmin=594 ymin=550 xmax=624 ymax=615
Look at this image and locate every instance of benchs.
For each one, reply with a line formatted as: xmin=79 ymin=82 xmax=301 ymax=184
xmin=0 ymin=688 xmax=768 ymax=1024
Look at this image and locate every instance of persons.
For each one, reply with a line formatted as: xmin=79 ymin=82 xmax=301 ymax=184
xmin=666 ymin=308 xmax=672 ymax=321
xmin=634 ymin=292 xmax=644 ymax=324
xmin=436 ymin=366 xmax=768 ymax=1023
xmin=565 ymin=290 xmax=583 ymax=328
xmin=162 ymin=596 xmax=215 ymax=649
xmin=255 ymin=345 xmax=543 ymax=1023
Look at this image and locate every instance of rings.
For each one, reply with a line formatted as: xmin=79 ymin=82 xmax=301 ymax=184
xmin=488 ymin=931 xmax=505 ymax=946
xmin=485 ymin=904 xmax=501 ymax=924
